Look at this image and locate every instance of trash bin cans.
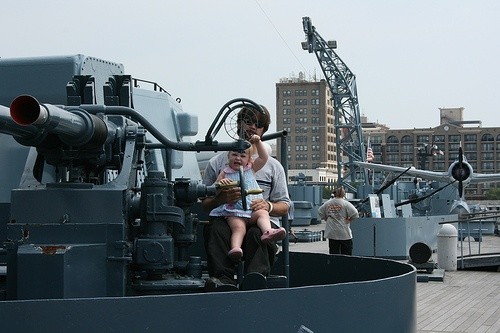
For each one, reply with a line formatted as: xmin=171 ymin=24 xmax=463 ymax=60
xmin=435 ymin=223 xmax=459 ymax=272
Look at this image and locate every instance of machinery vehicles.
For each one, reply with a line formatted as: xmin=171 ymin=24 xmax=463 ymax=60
xmin=0 ymin=53 xmax=417 ymax=333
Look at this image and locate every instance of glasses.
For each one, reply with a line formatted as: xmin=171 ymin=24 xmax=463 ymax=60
xmin=243 ymin=117 xmax=262 ymax=128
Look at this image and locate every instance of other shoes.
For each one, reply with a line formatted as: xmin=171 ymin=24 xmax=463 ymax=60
xmin=228 ymin=249 xmax=242 ymax=255
xmin=261 ymin=228 xmax=285 ymax=240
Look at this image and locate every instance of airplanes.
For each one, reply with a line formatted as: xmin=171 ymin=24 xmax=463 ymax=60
xmin=353 ymin=140 xmax=500 ymax=214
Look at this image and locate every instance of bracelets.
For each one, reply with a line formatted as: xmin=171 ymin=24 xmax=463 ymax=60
xmin=266 ymin=201 xmax=273 ymax=213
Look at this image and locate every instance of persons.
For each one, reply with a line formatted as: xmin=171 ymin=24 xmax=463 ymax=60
xmin=209 ymin=134 xmax=287 ymax=263
xmin=287 ymin=193 xmax=299 ymax=243
xmin=199 ymin=104 xmax=291 ymax=293
xmin=317 ymin=186 xmax=359 ymax=256
